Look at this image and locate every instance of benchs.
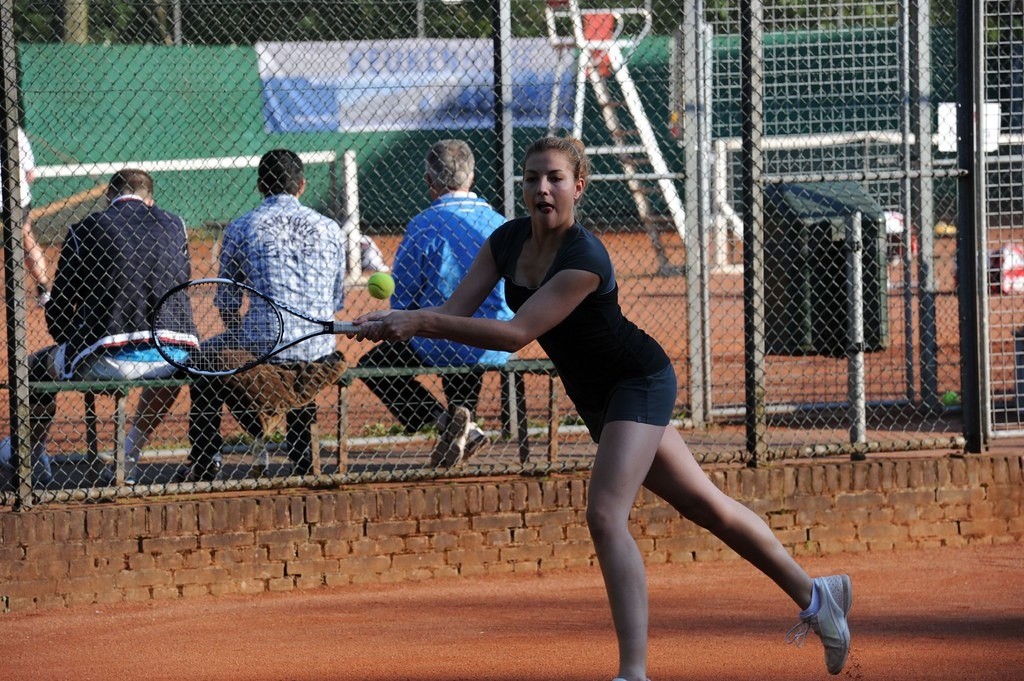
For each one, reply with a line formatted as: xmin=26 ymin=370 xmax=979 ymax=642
xmin=31 ymin=356 xmax=562 ymax=488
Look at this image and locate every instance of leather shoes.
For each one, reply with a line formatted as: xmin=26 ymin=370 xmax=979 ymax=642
xmin=177 ymin=462 xmax=221 ymax=481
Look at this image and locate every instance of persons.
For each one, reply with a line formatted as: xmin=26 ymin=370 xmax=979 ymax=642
xmin=17 ymin=124 xmax=52 ymax=307
xmin=171 ymin=149 xmax=346 ymax=484
xmin=1 ymin=169 xmax=199 ymax=485
xmin=346 ymin=136 xmax=851 ymax=681
xmin=356 ymin=139 xmax=516 ymax=469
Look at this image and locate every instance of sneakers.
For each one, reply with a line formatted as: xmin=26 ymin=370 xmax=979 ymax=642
xmin=102 ymin=458 xmax=137 ymax=485
xmin=800 ymin=575 xmax=851 ymax=674
xmin=463 ymin=424 xmax=487 ymax=464
xmin=431 ymin=408 xmax=467 ymax=469
xmin=1 ymin=435 xmax=51 ymax=481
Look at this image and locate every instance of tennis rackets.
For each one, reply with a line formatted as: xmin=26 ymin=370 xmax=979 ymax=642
xmin=149 ymin=279 xmax=395 ymax=376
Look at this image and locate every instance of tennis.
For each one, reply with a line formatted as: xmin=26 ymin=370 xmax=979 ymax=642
xmin=368 ymin=272 xmax=396 ymax=298
xmin=944 ymin=391 xmax=959 ymax=405
